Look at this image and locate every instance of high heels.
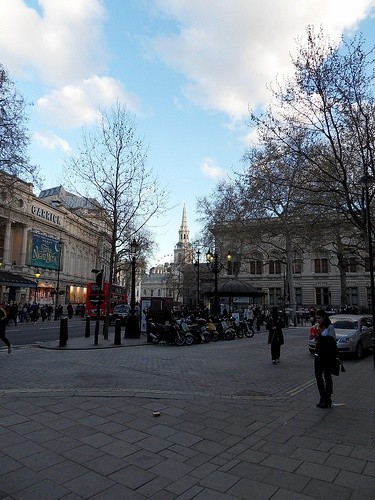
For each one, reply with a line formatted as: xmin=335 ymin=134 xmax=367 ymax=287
xmin=316 ymin=398 xmax=326 ymax=407
xmin=318 ymin=399 xmax=332 ymax=408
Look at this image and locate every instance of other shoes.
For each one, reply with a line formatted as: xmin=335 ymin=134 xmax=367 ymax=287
xmin=272 ymin=360 xmax=276 ymax=364
xmin=8 ymin=343 xmax=12 ymax=354
xmin=276 ymin=359 xmax=279 ymax=363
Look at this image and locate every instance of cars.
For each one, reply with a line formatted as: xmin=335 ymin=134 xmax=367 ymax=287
xmin=308 ymin=315 xmax=374 ymax=360
xmin=113 ymin=305 xmax=131 ymax=319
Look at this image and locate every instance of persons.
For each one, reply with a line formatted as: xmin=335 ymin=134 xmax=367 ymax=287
xmin=0 ymin=307 xmax=12 ymax=354
xmin=247 ymin=303 xmax=261 ymax=331
xmin=310 ymin=309 xmax=336 ymax=408
xmin=310 ymin=308 xmax=316 ymax=326
xmin=0 ymin=300 xmax=86 ymax=326
xmin=266 ymin=306 xmax=284 ymax=364
xmin=223 ymin=306 xmax=227 ymax=314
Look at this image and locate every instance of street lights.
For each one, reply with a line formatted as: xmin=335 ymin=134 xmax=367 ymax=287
xmin=124 ymin=237 xmax=141 ymax=339
xmin=56 ymin=238 xmax=65 ymax=308
xmin=197 ymin=248 xmax=201 ymax=305
xmin=33 ymin=269 xmax=41 ymax=304
xmin=358 ymin=171 xmax=374 ymax=334
xmin=281 ymin=255 xmax=286 ymax=314
xmin=207 ymin=246 xmax=233 ymax=311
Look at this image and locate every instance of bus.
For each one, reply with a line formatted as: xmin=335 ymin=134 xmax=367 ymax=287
xmin=86 ymin=281 xmax=128 ymax=319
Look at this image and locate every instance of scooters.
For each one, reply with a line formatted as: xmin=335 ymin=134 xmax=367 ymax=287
xmin=148 ymin=312 xmax=254 ymax=345
